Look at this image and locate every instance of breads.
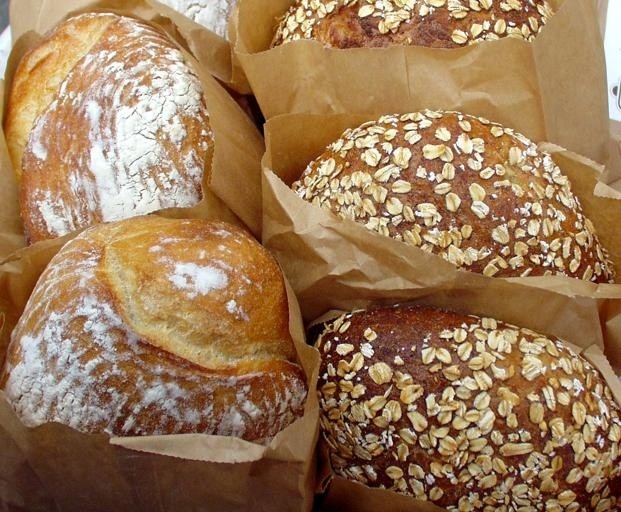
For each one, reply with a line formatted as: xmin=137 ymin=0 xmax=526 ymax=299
xmin=155 ymin=0 xmax=229 ymax=42
xmin=291 ymin=107 xmax=616 ymax=283
xmin=315 ymin=306 xmax=621 ymax=512
xmin=268 ymin=1 xmax=556 ymax=50
xmin=3 ymin=11 xmax=214 ymax=246
xmin=0 ymin=213 xmax=309 ymax=442
